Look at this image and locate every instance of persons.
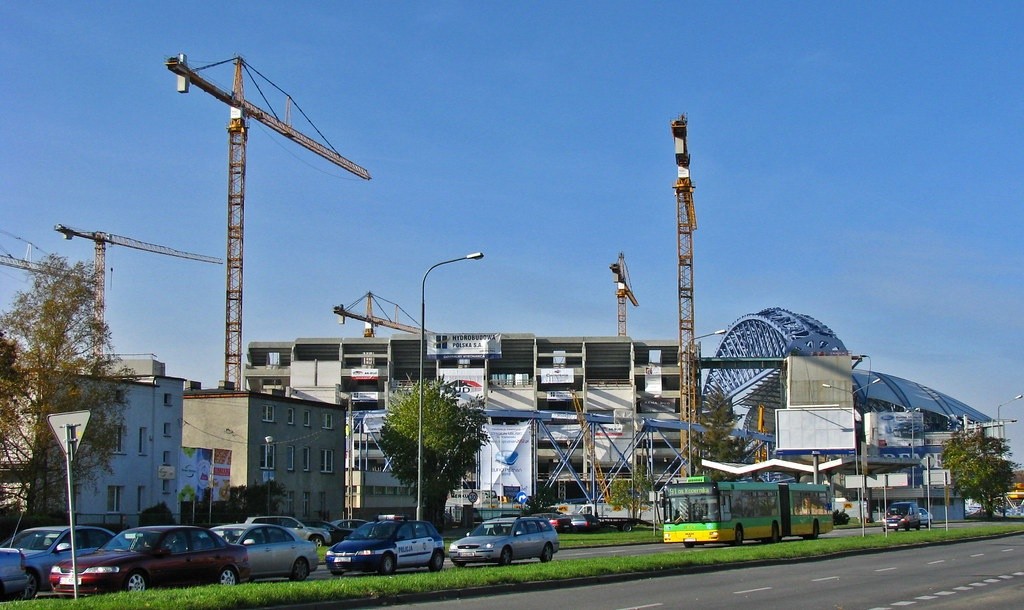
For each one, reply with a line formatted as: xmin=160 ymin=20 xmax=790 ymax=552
xmin=224 ymin=531 xmax=234 ymax=543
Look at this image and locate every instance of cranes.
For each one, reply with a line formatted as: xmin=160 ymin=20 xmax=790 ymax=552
xmin=669 ymin=111 xmax=699 ymax=477
xmin=165 ymin=53 xmax=371 ymax=386
xmin=334 ymin=292 xmax=428 ymax=339
xmin=56 ymin=224 xmax=222 ymax=379
xmin=610 ymin=252 xmax=639 ymax=335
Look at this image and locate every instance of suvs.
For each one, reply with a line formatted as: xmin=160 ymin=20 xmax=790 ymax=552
xmin=245 ymin=516 xmax=331 ymax=548
xmin=448 ymin=516 xmax=559 ymax=567
xmin=561 ymin=514 xmax=597 ymax=531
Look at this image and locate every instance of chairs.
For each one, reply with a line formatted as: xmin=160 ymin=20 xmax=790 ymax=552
xmin=493 ymin=526 xmax=504 ymax=536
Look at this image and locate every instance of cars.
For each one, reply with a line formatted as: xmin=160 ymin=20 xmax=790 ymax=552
xmin=49 ymin=524 xmax=250 ymax=597
xmin=0 ymin=548 xmax=30 ymax=601
xmin=327 ymin=519 xmax=371 ymax=542
xmin=531 ymin=513 xmax=572 ymax=532
xmin=207 ymin=523 xmax=319 ymax=582
xmin=0 ymin=526 xmax=134 ymax=599
xmin=918 ymin=508 xmax=934 ymax=527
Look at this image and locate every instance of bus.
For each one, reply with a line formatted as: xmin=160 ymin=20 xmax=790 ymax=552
xmin=659 ymin=475 xmax=835 ymax=549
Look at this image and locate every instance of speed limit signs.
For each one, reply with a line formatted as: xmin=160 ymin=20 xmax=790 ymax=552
xmin=468 ymin=492 xmax=479 ymax=503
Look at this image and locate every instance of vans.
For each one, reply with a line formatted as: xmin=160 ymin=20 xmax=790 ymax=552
xmin=884 ymin=502 xmax=920 ymax=532
xmin=325 ymin=520 xmax=445 ymax=574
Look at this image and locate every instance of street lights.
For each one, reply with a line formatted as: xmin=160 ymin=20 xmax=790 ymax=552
xmin=688 ymin=330 xmax=727 ymax=475
xmin=265 ymin=434 xmax=273 ymax=515
xmin=823 ymin=377 xmax=881 ymax=538
xmin=906 ymin=407 xmax=921 ymax=489
xmin=416 ymin=253 xmax=485 ymax=522
xmin=998 ymin=395 xmax=1022 ymax=516
